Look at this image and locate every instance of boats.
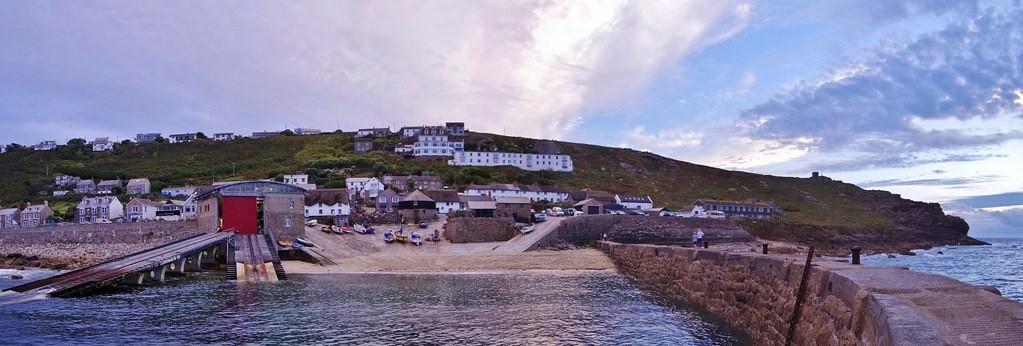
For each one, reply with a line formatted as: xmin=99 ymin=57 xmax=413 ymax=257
xmin=408 ymin=221 xmax=414 ymax=227
xmin=537 ymin=214 xmax=547 ymax=222
xmin=292 ymin=242 xmax=302 ymax=249
xmin=419 ymin=221 xmax=427 ymax=228
xmin=363 ymin=224 xmax=375 ymax=234
xmin=353 ymin=223 xmax=366 ymax=233
xmin=432 ymin=232 xmax=440 ymax=242
xmin=279 ymin=240 xmax=294 ymax=247
xmin=297 ymin=237 xmax=313 ymax=247
xmin=411 ymin=232 xmax=423 ymax=246
xmin=342 ymin=224 xmax=352 ymax=233
xmin=331 ymin=225 xmax=344 ymax=234
xmin=322 ymin=226 xmax=331 ymax=234
xmin=306 ymin=219 xmax=317 ymax=227
xmin=520 ymin=222 xmax=535 ymax=234
xmin=394 ymin=229 xmax=408 ymax=243
xmin=384 ymin=232 xmax=394 ymax=243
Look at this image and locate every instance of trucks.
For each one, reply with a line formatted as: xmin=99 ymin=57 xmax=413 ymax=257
xmin=563 ymin=208 xmax=577 ymax=216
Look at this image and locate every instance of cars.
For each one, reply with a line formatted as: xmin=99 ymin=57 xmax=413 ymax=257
xmin=616 ymin=210 xmax=625 ymax=215
xmin=80 ymin=221 xmax=91 ymax=225
xmin=664 ymin=212 xmax=675 ymax=217
xmin=40 ymin=220 xmax=62 ymax=226
xmin=543 ymin=209 xmax=552 ymax=215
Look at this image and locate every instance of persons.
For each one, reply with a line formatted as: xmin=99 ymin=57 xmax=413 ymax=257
xmin=697 ymin=229 xmax=704 ymax=248
xmin=690 ymin=232 xmax=697 ymax=247
xmin=537 ymin=242 xmax=540 ymax=252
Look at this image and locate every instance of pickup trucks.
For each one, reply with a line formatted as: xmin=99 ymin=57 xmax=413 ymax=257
xmin=630 ymin=210 xmax=645 ymax=216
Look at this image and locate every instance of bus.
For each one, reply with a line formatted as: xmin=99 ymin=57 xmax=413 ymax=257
xmin=706 ymin=211 xmax=725 ymax=220
xmin=155 ymin=210 xmax=181 ymax=222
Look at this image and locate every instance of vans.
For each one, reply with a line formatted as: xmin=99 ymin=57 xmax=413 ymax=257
xmin=96 ymin=218 xmax=113 ymax=223
xmin=552 ymin=207 xmax=564 ymax=217
